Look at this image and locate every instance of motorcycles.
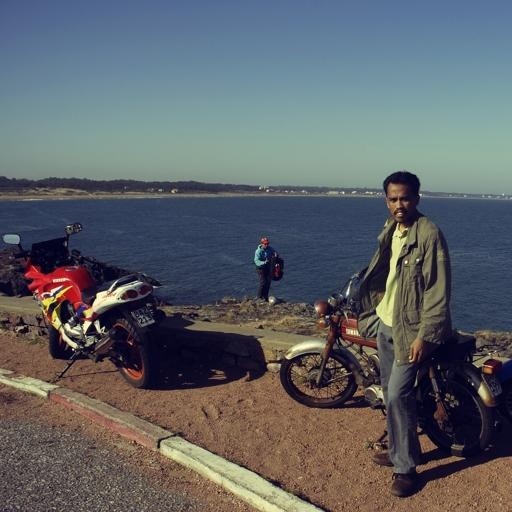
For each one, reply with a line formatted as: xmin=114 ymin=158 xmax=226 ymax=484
xmin=277 ymin=265 xmax=504 ymax=460
xmin=1 ymin=221 xmax=168 ymax=390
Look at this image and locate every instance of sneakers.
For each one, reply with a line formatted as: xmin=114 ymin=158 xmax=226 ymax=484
xmin=374 ymin=453 xmax=393 ymax=466
xmin=391 ymin=471 xmax=419 ymax=497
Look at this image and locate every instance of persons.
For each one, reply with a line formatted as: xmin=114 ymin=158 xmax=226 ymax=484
xmin=253 ymin=237 xmax=280 ymax=301
xmin=358 ymin=170 xmax=454 ymax=499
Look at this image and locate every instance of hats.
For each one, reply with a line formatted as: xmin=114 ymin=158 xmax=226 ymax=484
xmin=260 ymin=237 xmax=268 ymax=244
